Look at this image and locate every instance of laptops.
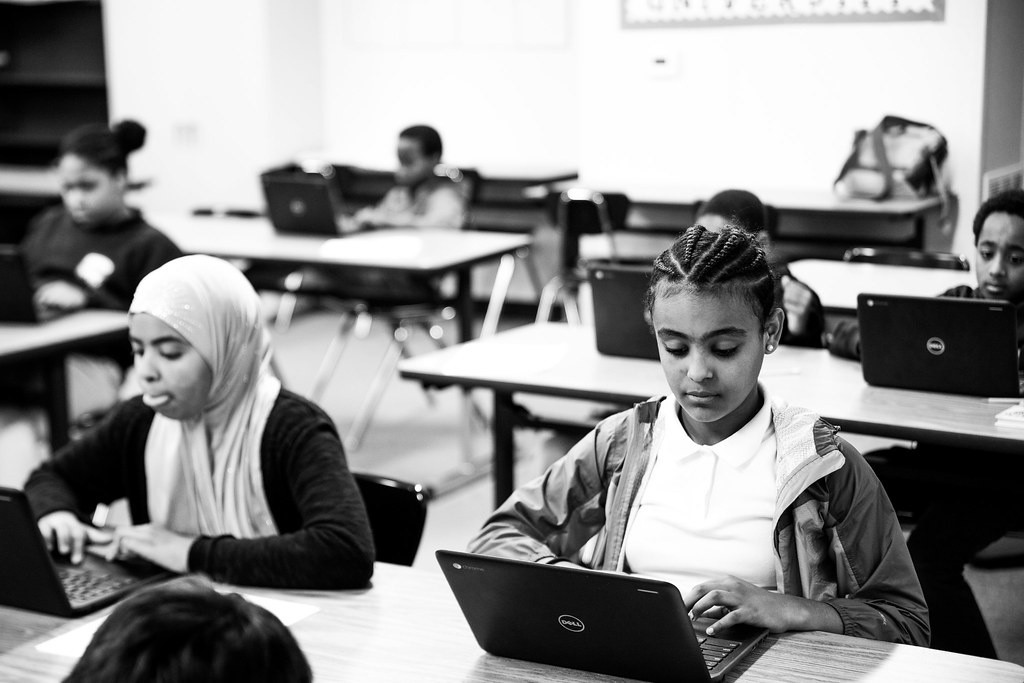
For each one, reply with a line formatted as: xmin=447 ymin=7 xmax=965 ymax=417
xmin=435 ymin=549 xmax=770 ymax=683
xmin=587 ymin=264 xmax=659 ymax=360
xmin=0 ymin=249 xmax=78 ymax=324
xmin=1 ymin=488 xmax=178 ymax=619
xmin=261 ymin=174 xmax=374 ymax=237
xmin=858 ymin=294 xmax=1024 ymax=399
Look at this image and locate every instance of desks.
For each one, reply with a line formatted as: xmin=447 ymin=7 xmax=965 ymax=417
xmin=0 ymin=151 xmax=1022 ymax=683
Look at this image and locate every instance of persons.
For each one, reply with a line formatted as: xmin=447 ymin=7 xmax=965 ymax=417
xmin=462 ymin=223 xmax=932 ymax=649
xmin=820 ymin=189 xmax=1024 ymax=660
xmin=19 ymin=253 xmax=376 ymax=590
xmin=15 ymin=117 xmax=185 ymax=313
xmin=57 ymin=589 xmax=312 ymax=683
xmin=349 ymin=125 xmax=470 ymax=317
xmin=694 ymin=188 xmax=829 ymax=349
